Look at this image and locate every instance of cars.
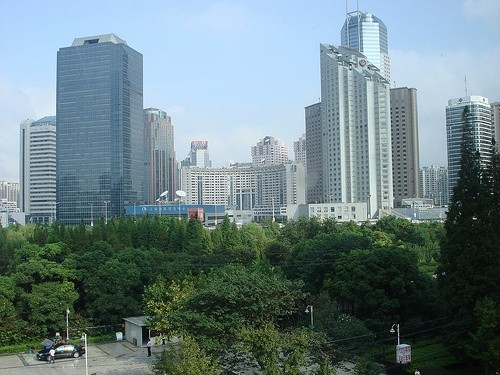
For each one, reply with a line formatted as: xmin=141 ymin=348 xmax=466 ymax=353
xmin=37 ymin=337 xmax=85 ymax=361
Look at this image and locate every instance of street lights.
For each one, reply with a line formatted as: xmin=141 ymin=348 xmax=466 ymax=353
xmin=132 ymin=202 xmax=137 ymax=218
xmin=305 ymin=305 xmax=313 ymax=329
xmin=80 ymin=332 xmax=89 ymax=375
xmin=174 ymin=190 xmax=186 ymax=221
xmin=390 ymin=323 xmax=400 ymax=345
xmin=104 ymin=201 xmax=110 ymax=224
xmin=66 ymin=308 xmax=71 ymax=339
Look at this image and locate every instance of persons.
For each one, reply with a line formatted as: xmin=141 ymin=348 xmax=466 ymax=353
xmin=49 ymin=347 xmax=55 ymax=364
xmin=56 ymin=332 xmax=62 ymax=344
xmin=147 ymin=339 xmax=151 ymax=357
